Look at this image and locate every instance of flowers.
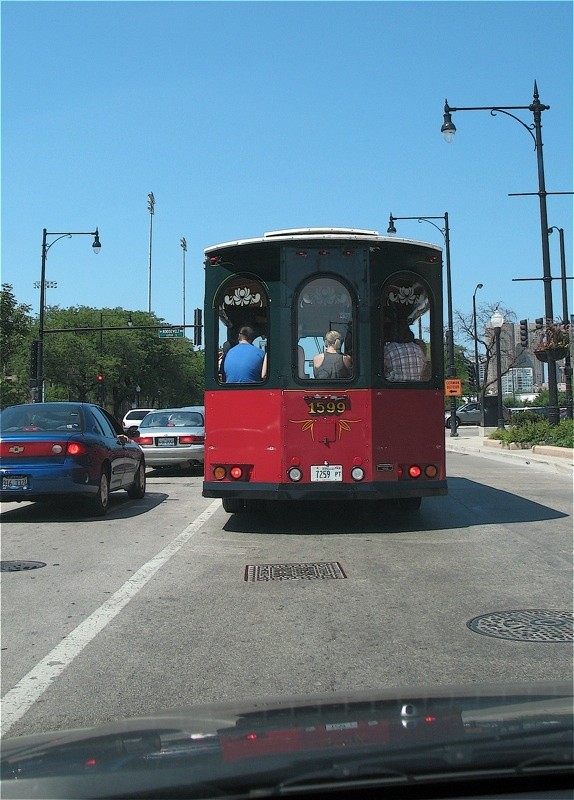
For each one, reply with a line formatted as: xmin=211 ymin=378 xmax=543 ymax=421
xmin=535 ymin=323 xmax=571 ymax=350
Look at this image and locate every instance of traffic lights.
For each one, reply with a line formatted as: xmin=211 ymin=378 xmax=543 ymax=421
xmin=520 ymin=319 xmax=529 ymax=348
xmin=98 ymin=375 xmax=103 ymax=394
xmin=535 ymin=318 xmax=544 ymax=344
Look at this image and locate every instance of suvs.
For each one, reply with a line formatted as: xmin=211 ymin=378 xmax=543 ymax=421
xmin=121 ymin=408 xmax=157 ymax=436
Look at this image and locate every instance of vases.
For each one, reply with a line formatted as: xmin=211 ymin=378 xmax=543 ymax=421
xmin=534 ymin=348 xmax=568 ymax=362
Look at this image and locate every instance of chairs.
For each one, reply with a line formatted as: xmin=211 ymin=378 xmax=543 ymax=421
xmin=184 ymin=416 xmax=201 ymax=426
xmin=11 ymin=412 xmax=80 ymax=430
xmin=151 ymin=417 xmax=169 ymax=426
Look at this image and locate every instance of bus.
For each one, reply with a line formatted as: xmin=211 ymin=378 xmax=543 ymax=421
xmin=197 ymin=226 xmax=448 ymax=513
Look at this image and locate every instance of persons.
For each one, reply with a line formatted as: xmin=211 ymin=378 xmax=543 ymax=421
xmin=313 ymin=330 xmax=352 ymax=379
xmin=384 ymin=312 xmax=428 ymax=383
xmin=218 ymin=326 xmax=308 ymax=384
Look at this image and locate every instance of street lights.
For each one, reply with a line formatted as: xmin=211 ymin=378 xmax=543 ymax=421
xmin=547 ymin=225 xmax=573 ymax=418
xmin=384 ymin=211 xmax=462 ymax=439
xmin=99 ymin=311 xmax=132 ymax=401
xmin=472 ymin=283 xmax=484 ymax=400
xmin=147 ymin=191 xmax=155 ymax=314
xmin=37 ymin=227 xmax=102 ymax=403
xmin=441 ymin=78 xmax=561 ymax=431
xmin=180 ymin=236 xmax=187 ymax=338
xmin=490 ymin=306 xmax=510 ymax=434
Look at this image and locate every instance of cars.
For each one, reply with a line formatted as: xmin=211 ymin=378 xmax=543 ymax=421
xmin=135 ymin=406 xmax=206 ymax=471
xmin=443 ymin=402 xmax=483 ymax=429
xmin=1 ymin=402 xmax=148 ymax=512
xmin=1 ymin=681 xmax=573 ymax=800
